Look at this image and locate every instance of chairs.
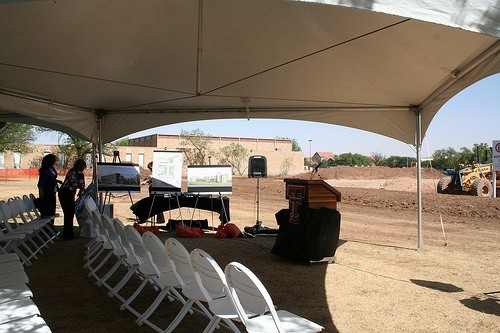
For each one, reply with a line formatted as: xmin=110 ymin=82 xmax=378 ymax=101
xmin=0 ymin=193 xmax=61 ymax=333
xmin=84 ymin=194 xmax=325 ymax=333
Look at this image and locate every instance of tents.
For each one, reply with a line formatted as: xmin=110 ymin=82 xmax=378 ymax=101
xmin=0 ymin=0 xmax=500 ymax=249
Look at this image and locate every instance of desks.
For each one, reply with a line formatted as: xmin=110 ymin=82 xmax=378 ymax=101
xmin=130 ymin=194 xmax=230 ymax=233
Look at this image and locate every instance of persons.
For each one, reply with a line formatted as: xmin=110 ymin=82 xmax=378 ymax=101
xmin=37 ymin=154 xmax=64 ymax=224
xmin=58 ymin=159 xmax=86 ymax=240
xmin=146 ymin=161 xmax=165 ymax=223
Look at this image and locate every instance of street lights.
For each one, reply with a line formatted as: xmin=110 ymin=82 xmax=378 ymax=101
xmin=208 ymin=155 xmax=211 ymax=165
xmin=308 ymin=139 xmax=312 ymax=167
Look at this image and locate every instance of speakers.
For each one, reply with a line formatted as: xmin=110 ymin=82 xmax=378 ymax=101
xmin=248 ymin=155 xmax=267 ymax=178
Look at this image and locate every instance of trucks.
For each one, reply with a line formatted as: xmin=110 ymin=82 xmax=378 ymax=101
xmin=247 ymin=155 xmax=268 ymax=178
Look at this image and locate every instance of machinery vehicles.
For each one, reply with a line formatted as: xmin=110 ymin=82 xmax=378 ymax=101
xmin=436 ymin=146 xmax=500 ymax=198
